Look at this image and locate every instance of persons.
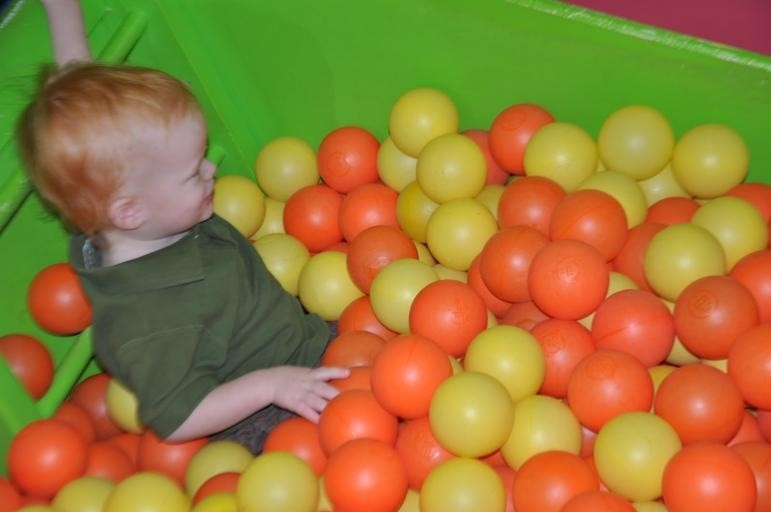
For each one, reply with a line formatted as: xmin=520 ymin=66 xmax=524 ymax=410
xmin=14 ymin=3 xmax=356 ymax=462
